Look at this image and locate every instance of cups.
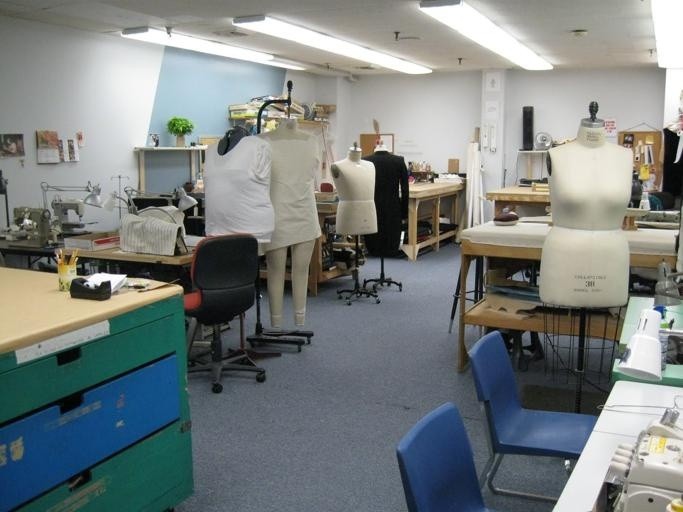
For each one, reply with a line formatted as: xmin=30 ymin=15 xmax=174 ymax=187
xmin=57 ymin=264 xmax=77 ymax=291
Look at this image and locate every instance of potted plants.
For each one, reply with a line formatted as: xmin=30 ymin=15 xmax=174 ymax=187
xmin=167 ymin=115 xmax=195 ymax=147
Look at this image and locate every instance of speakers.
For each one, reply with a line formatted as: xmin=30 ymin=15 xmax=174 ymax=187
xmin=522 ymin=106 xmax=534 ymax=150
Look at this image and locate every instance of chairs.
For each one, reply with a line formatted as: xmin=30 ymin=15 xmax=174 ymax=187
xmin=184 ymin=233 xmax=266 ymax=393
xmin=128 ymin=198 xmax=205 ymax=237
xmin=395 ymin=330 xmax=599 ymax=512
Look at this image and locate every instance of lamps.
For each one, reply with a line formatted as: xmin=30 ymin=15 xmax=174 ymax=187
xmin=232 ymin=16 xmax=432 ymax=76
xmin=120 ymin=26 xmax=305 ymax=71
xmin=124 ymin=186 xmax=199 ymax=215
xmin=420 ymin=1 xmax=554 ymax=71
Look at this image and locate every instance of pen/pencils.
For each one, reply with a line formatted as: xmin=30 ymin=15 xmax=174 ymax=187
xmin=54 ymin=248 xmax=79 ymax=266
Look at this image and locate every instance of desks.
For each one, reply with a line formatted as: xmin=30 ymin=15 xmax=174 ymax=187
xmin=0 ymin=266 xmax=196 ymax=511
xmin=486 ymin=185 xmax=552 ymax=219
xmin=0 ymin=233 xmax=207 ymax=273
xmin=458 ymin=216 xmax=683 ymax=372
xmin=550 ymin=295 xmax=683 ymax=512
xmin=402 ymin=179 xmax=467 ymax=260
xmin=259 ymin=199 xmax=356 ymax=298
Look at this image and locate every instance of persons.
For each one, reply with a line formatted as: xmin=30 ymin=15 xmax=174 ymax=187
xmin=537 ymin=120 xmax=634 ymax=308
xmin=203 ymin=128 xmax=276 ymax=241
xmin=362 ymin=150 xmax=408 ymax=250
xmin=256 ymin=119 xmax=318 ymax=329
xmin=330 ymin=147 xmax=378 ymax=238
xmin=2 ymin=137 xmax=21 ymax=156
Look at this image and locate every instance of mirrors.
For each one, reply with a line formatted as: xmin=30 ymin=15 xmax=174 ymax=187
xmin=360 ymin=134 xmax=394 ymax=159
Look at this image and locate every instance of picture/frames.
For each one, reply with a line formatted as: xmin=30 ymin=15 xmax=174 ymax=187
xmin=64 ymin=137 xmax=79 ymax=162
